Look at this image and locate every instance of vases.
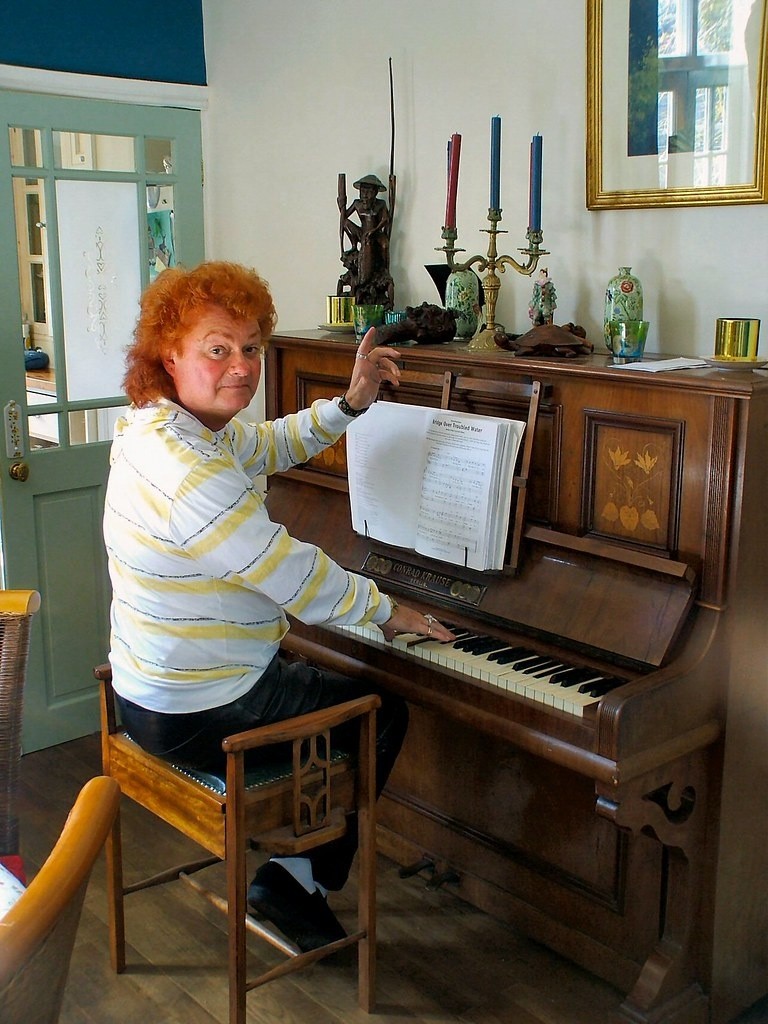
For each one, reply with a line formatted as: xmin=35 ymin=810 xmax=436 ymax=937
xmin=603 ymin=267 xmax=644 ymax=355
xmin=445 ymin=263 xmax=479 ymax=342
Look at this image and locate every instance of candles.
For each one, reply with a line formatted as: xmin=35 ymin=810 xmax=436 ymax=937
xmin=528 ymin=131 xmax=542 ymax=234
xmin=491 ymin=113 xmax=501 ymax=213
xmin=444 ymin=132 xmax=462 ymax=229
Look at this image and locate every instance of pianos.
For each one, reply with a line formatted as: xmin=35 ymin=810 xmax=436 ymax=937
xmin=260 ymin=327 xmax=768 ymax=1024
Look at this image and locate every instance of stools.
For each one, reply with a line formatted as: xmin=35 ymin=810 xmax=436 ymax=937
xmin=91 ymin=664 xmax=381 ymax=1024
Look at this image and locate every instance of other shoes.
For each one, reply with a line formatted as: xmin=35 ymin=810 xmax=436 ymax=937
xmin=247 ymin=861 xmax=346 ymax=964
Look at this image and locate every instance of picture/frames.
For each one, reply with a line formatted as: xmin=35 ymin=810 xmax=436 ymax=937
xmin=583 ymin=0 xmax=768 ymax=213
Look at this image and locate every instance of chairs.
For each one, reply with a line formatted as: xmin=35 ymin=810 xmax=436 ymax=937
xmin=0 ymin=589 xmax=121 ymax=1024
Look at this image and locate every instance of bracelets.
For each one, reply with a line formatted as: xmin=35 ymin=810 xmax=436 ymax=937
xmin=339 ymin=393 xmax=369 ymax=417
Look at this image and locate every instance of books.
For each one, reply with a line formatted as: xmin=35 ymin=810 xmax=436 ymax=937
xmin=345 ymin=399 xmax=526 ymax=572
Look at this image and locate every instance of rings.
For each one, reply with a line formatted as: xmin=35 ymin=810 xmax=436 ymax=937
xmin=356 ymin=352 xmax=368 ymax=360
xmin=376 ymin=363 xmax=381 ymax=369
xmin=423 ymin=614 xmax=437 ymax=626
xmin=427 ymin=627 xmax=433 ymax=636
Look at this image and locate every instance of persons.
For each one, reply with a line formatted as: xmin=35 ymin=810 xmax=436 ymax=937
xmin=103 ymin=260 xmax=457 ymax=964
xmin=528 ymin=268 xmax=558 ymax=326
xmin=337 ymin=175 xmax=391 ymax=267
